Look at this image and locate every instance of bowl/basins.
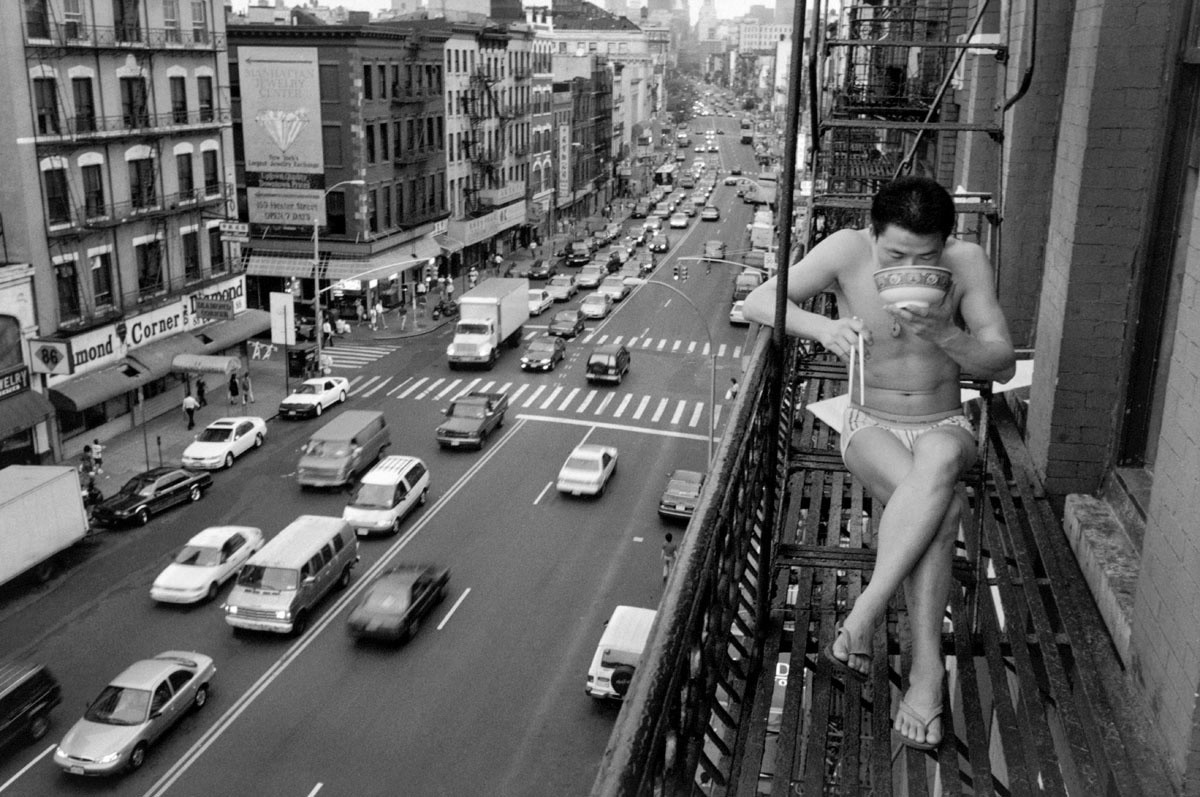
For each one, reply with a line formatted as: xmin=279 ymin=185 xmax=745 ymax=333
xmin=872 ymin=265 xmax=953 ymax=309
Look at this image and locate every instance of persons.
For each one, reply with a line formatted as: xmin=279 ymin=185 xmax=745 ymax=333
xmin=356 ymin=299 xmax=388 ymax=331
xmin=469 ymin=266 xmax=478 ymax=289
xmin=494 ymin=253 xmax=504 ymax=274
xmin=398 ymin=304 xmax=407 ymax=330
xmin=295 ymin=318 xmax=334 ymax=373
xmin=416 ymin=271 xmax=454 ymax=301
xmin=529 ymin=238 xmax=537 ymax=260
xmin=182 ymin=373 xmax=208 ymax=430
xmin=743 ymin=176 xmax=1017 ymax=750
xmin=79 ymin=439 xmax=107 ymax=489
xmin=229 ymin=371 xmax=255 ymax=407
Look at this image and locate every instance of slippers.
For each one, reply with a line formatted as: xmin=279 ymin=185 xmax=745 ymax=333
xmin=891 ymin=700 xmax=944 ymax=750
xmin=822 ymin=621 xmax=876 ymax=681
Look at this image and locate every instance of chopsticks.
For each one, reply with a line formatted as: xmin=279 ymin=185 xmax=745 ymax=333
xmin=847 ymin=316 xmax=868 ymax=408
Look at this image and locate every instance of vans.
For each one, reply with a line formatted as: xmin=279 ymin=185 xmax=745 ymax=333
xmin=227 ymin=512 xmax=360 ymax=637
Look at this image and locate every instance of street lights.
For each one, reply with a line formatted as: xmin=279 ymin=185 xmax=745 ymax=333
xmin=312 ymin=179 xmax=436 ymax=376
xmin=677 ymin=176 xmax=776 ymax=284
xmin=623 ymin=277 xmax=715 ymax=471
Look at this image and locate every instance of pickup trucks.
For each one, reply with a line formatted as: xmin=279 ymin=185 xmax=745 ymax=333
xmin=432 ymin=392 xmax=510 ymax=451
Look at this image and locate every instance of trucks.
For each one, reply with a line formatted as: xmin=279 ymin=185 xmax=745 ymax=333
xmin=447 ymin=277 xmax=530 ymax=370
xmin=1 ymin=464 xmax=84 ymax=596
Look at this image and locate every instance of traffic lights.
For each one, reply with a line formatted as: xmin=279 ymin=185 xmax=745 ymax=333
xmin=432 ymin=266 xmax=439 ymax=281
xmin=423 ymin=265 xmax=434 ymax=285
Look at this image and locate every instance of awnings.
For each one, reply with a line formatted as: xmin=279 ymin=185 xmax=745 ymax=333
xmin=48 ymin=308 xmax=271 ymax=413
xmin=0 ymin=390 xmax=56 ymax=440
xmin=245 ymin=237 xmax=445 ymax=280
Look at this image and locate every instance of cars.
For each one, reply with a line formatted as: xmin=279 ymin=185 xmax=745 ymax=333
xmin=556 ymin=443 xmax=620 ymax=498
xmin=147 ymin=526 xmax=267 ymax=607
xmin=279 ymin=376 xmax=351 ymax=421
xmin=549 ymin=309 xmax=586 ymax=340
xmin=51 ymin=650 xmax=218 ymax=778
xmin=731 ymin=300 xmax=750 ymax=325
xmin=528 ymin=89 xmax=783 ymax=318
xmin=699 ymin=239 xmax=729 ymax=263
xmin=346 ymin=563 xmax=452 ymax=647
xmin=179 ymin=415 xmax=268 ymax=471
xmin=519 ymin=335 xmax=568 ymax=372
xmin=660 ymin=469 xmax=706 ymax=520
xmin=92 ymin=466 xmax=215 ymax=530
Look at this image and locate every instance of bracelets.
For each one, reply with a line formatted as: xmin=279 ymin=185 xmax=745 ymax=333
xmin=937 ymin=330 xmax=960 ymax=345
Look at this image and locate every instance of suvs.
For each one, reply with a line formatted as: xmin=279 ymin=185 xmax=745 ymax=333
xmin=338 ymin=455 xmax=431 ymax=536
xmin=295 ymin=409 xmax=395 ymax=491
xmin=584 ymin=342 xmax=632 ymax=387
xmin=0 ymin=659 xmax=64 ymax=751
xmin=586 ymin=605 xmax=667 ymax=704
